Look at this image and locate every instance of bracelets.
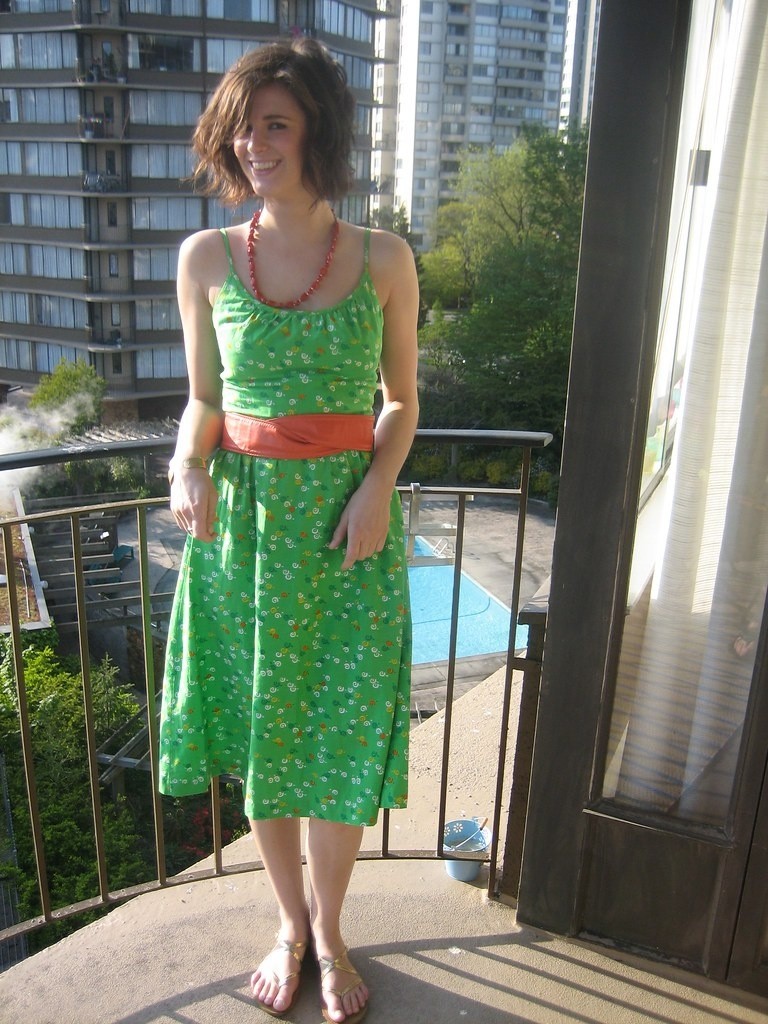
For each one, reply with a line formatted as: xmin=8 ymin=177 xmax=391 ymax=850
xmin=168 ymin=458 xmax=207 ymax=485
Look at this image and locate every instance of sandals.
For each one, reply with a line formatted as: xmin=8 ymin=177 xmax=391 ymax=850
xmin=312 ymin=942 xmax=369 ymax=1024
xmin=251 ymin=929 xmax=306 ymax=1015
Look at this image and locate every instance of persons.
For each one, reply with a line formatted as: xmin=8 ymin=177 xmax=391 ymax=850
xmin=157 ymin=38 xmax=420 ymax=1023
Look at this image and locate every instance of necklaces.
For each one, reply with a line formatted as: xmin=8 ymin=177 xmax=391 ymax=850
xmin=247 ymin=206 xmax=340 ymax=309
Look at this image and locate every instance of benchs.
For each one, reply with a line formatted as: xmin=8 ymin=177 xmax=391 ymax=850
xmin=108 ymin=545 xmax=134 ymax=569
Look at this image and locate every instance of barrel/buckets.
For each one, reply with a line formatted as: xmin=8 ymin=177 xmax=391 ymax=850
xmin=443 ymin=816 xmax=492 ymax=881
